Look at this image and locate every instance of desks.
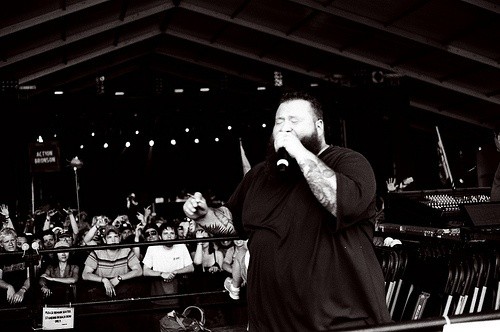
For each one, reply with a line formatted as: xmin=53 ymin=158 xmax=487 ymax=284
xmin=0 ymin=271 xmax=247 ymax=332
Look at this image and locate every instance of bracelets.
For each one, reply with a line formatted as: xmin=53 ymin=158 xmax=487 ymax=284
xmin=20 ymin=287 xmax=27 ymax=291
xmin=5 ymin=216 xmax=10 ymax=219
xmin=95 ymin=224 xmax=99 ymax=229
xmin=46 ymin=217 xmax=50 ymax=222
xmin=160 ymin=272 xmax=163 ymax=276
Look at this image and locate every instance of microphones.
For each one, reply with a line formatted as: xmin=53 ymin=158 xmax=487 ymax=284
xmin=277 ymin=145 xmax=289 ymax=171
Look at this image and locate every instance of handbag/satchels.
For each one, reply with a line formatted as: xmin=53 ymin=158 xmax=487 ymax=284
xmin=159 ymin=306 xmax=211 ymax=332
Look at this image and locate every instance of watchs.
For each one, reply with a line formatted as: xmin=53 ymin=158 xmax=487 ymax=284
xmin=117 ymin=275 xmax=122 ymax=281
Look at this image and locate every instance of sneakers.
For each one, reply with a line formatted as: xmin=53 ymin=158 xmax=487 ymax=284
xmin=224 ymin=277 xmax=240 ymax=299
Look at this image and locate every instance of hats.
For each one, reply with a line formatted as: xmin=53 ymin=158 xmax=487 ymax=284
xmin=54 ymin=241 xmax=70 ymax=248
xmin=105 ymin=226 xmax=118 ymax=234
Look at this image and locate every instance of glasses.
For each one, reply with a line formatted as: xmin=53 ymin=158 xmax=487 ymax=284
xmin=145 ymin=231 xmax=156 ymax=237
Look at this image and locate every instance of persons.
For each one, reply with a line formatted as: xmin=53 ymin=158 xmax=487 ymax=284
xmin=182 ymin=91 xmax=395 ymax=332
xmin=0 ymin=125 xmax=500 ymax=315
